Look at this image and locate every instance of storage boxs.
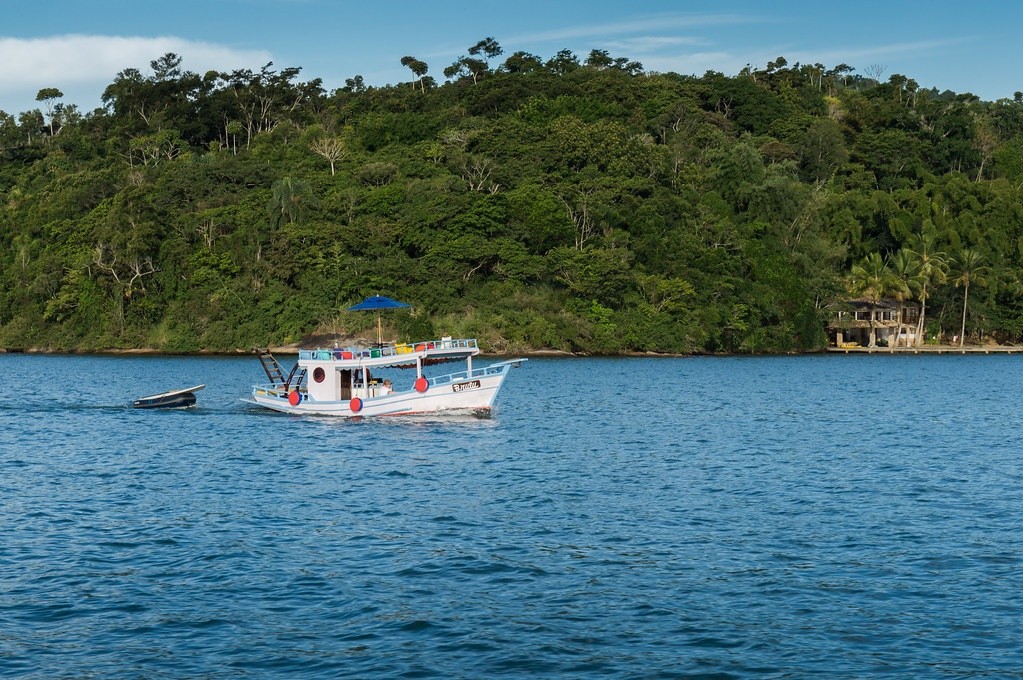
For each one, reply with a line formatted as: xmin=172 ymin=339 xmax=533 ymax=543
xmin=299 ymin=336 xmax=452 ymax=361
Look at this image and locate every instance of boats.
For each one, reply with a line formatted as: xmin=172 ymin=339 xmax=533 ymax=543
xmin=239 ymin=336 xmax=529 ymax=420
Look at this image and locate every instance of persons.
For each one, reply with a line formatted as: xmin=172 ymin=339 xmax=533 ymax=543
xmin=366 ymin=368 xmax=371 ymax=382
xmin=379 ymin=380 xmax=400 ymax=396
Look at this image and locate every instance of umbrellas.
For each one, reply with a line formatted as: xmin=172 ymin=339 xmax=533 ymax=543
xmin=348 ymin=294 xmax=410 ymax=342
